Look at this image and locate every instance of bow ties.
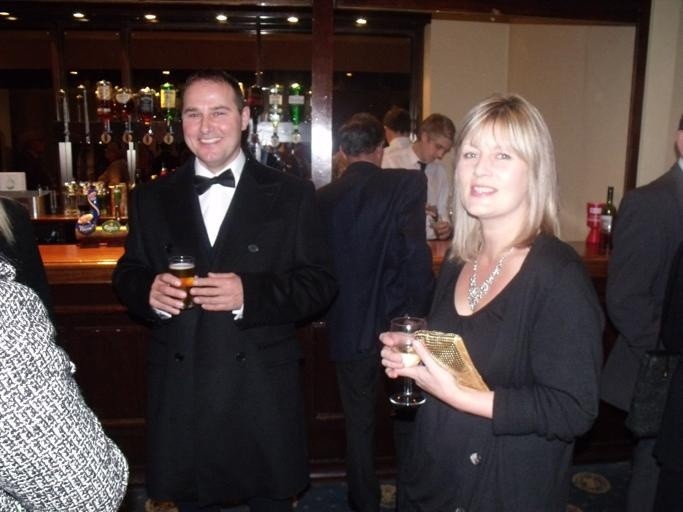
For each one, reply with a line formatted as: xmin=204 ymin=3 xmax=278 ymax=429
xmin=192 ymin=168 xmax=234 ymax=195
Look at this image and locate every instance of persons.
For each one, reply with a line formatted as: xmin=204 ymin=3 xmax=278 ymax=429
xmin=114 ymin=72 xmax=339 ymax=511
xmin=384 ymin=112 xmax=452 ymax=241
xmin=648 ymin=239 xmax=683 ymax=510
xmin=313 ymin=114 xmax=434 ymax=510
xmin=378 ymin=96 xmax=605 ymax=511
xmin=598 ymin=102 xmax=682 ymax=511
xmin=0 ymin=196 xmax=55 ymax=311
xmin=381 ymin=106 xmax=413 ymax=155
xmin=98 ymin=142 xmax=126 ymax=184
xmin=0 ymin=255 xmax=131 ymax=511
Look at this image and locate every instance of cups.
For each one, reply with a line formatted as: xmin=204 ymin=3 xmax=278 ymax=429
xmin=167 ymin=255 xmax=197 ymax=310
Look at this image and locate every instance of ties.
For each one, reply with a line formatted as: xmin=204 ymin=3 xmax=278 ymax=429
xmin=417 ymin=161 xmax=426 ymax=173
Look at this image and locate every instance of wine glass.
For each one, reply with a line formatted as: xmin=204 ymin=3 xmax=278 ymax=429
xmin=388 ymin=316 xmax=428 ymax=406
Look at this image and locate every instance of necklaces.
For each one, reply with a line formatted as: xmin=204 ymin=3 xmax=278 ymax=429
xmin=466 ymin=245 xmax=526 ymax=307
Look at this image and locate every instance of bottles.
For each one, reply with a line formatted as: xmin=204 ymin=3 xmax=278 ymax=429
xmin=245 ymin=82 xmax=306 ymax=174
xmin=93 ymin=79 xmax=177 ymax=134
xmin=134 ymin=159 xmax=178 ymax=185
xmin=599 ymin=187 xmax=617 ymax=249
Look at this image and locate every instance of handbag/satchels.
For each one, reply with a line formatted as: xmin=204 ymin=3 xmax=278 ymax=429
xmin=628 ymin=351 xmax=678 ymax=438
xmin=414 ymin=331 xmax=489 ymax=392
